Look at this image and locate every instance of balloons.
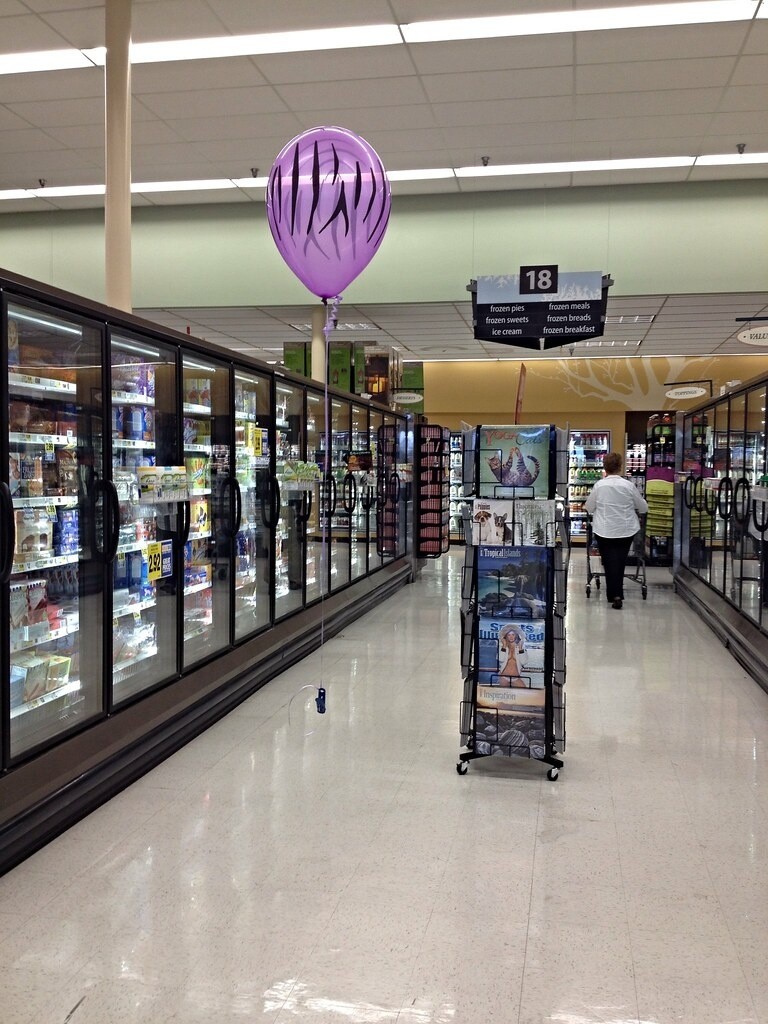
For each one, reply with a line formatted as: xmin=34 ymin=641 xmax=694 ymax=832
xmin=268 ymin=124 xmax=391 ymax=303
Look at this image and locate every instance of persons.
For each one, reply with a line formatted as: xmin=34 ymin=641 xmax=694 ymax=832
xmin=581 ymin=453 xmax=648 ymax=611
xmin=748 ymin=460 xmax=768 ymax=608
xmin=496 ymin=625 xmax=529 ymax=687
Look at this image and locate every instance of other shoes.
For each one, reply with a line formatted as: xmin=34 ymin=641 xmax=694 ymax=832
xmin=612 ymin=599 xmax=623 ymax=608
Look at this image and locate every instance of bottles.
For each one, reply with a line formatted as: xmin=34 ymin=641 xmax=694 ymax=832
xmin=450 ymin=436 xmax=462 ymax=451
xmin=595 ymin=454 xmax=605 ymax=462
xmin=450 ymin=484 xmax=464 ymax=498
xmin=570 ymin=436 xmax=607 ymax=446
xmin=450 ymin=468 xmax=462 ymax=484
xmin=450 ymin=499 xmax=466 ymax=514
xmin=570 ymin=467 xmax=602 ymax=480
xmin=570 ymin=454 xmax=586 ymax=462
xmin=450 ymin=452 xmax=462 ymax=468
xmin=448 ymin=517 xmax=459 ymax=531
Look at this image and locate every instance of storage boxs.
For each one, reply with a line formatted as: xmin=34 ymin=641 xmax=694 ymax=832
xmin=392 ymin=348 xmax=398 ymax=394
xmin=364 ymin=345 xmax=393 ymax=406
xmin=402 ymin=361 xmax=424 ymax=415
xmin=397 ymin=353 xmax=404 ymax=392
xmin=306 ymin=341 xmax=326 ymax=378
xmin=354 ymin=340 xmax=377 ymax=394
xmin=284 ymin=341 xmax=306 ymax=377
xmin=327 ymin=341 xmax=353 ymax=393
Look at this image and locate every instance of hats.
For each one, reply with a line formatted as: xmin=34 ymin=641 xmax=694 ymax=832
xmin=499 ymin=623 xmax=526 ymax=641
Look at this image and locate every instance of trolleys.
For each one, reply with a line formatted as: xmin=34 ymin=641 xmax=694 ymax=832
xmin=584 ymin=509 xmax=649 ymax=600
